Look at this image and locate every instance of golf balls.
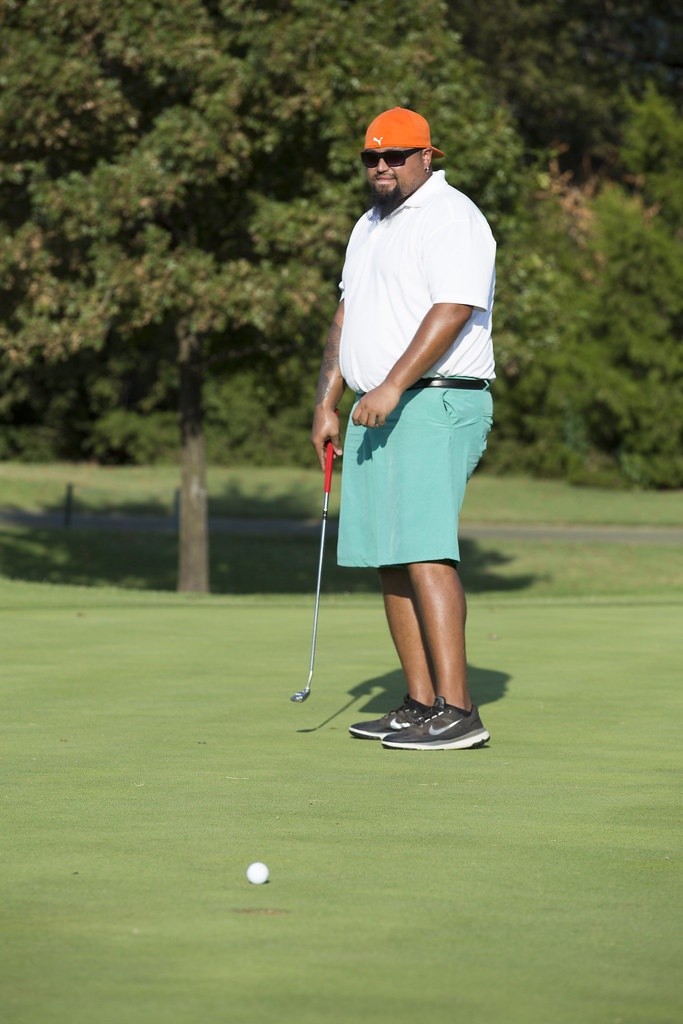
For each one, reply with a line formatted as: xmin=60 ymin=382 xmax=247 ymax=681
xmin=247 ymin=863 xmax=269 ymax=885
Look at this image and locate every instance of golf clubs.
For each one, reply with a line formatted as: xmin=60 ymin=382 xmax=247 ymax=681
xmin=289 ymin=440 xmax=333 ymax=704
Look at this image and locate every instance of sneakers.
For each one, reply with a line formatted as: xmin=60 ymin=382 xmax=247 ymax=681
xmin=346 ymin=695 xmax=448 ymax=738
xmin=381 ymin=692 xmax=490 ymax=751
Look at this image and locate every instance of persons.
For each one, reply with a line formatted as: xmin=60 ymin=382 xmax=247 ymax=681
xmin=311 ymin=107 xmax=496 ymax=753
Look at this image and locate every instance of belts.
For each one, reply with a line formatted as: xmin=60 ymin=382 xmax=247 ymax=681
xmin=406 ymin=375 xmax=490 ymax=391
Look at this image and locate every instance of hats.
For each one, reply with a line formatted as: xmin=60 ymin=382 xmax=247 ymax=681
xmin=364 ymin=106 xmax=445 ymax=160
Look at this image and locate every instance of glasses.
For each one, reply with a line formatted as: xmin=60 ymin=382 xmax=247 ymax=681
xmin=360 ymin=145 xmax=426 ymax=168
